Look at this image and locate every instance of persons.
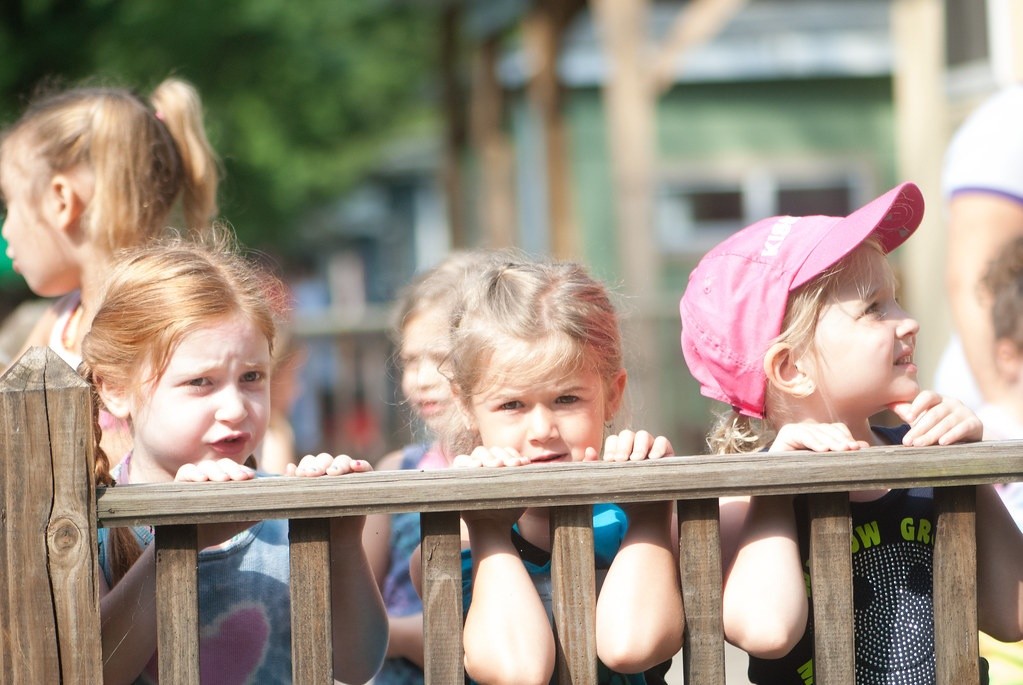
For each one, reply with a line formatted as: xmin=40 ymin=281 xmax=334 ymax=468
xmin=0 ymin=76 xmax=295 ymax=483
xmin=363 ymin=260 xmax=486 ymax=685
xmin=680 ymin=179 xmax=1023 ymax=685
xmin=934 ymin=75 xmax=1023 ymax=539
xmin=441 ymin=259 xmax=685 ymax=685
xmin=71 ymin=243 xmax=389 ymax=685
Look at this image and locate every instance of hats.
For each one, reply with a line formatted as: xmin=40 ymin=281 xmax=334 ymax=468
xmin=680 ymin=180 xmax=925 ymax=417
